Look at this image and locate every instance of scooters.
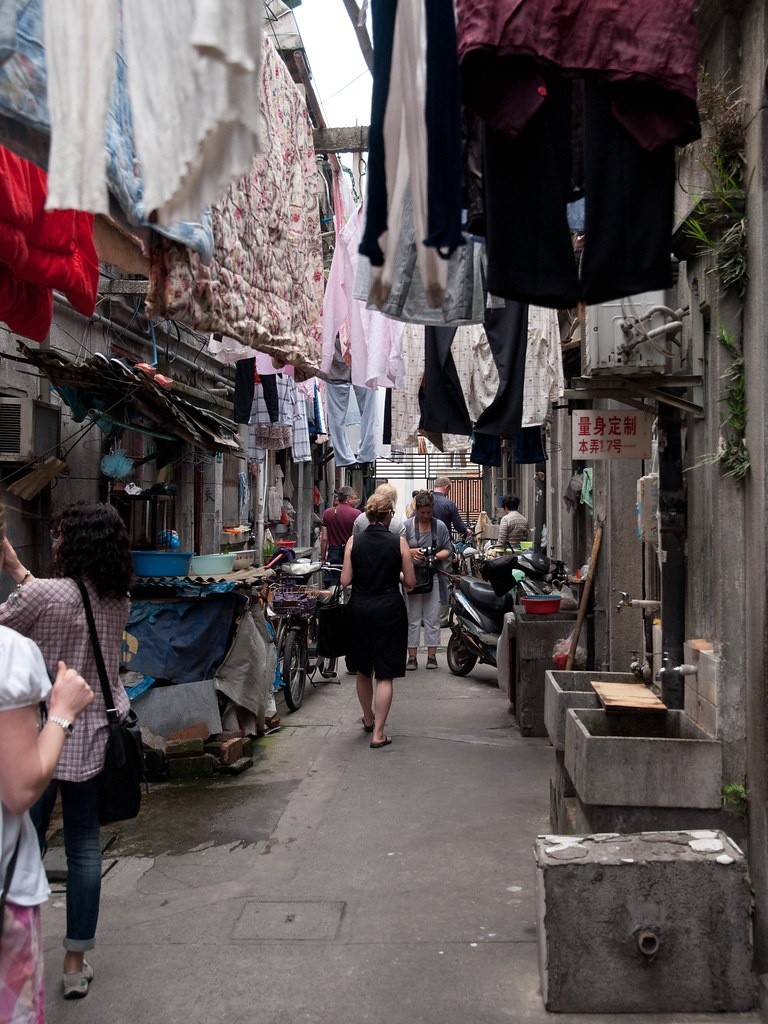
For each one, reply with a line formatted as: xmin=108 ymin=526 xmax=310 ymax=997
xmin=444 ymin=539 xmax=571 ymax=677
xmin=269 ymin=551 xmax=347 ymax=716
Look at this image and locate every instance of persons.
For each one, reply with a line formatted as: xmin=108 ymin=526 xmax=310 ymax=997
xmin=0 ymin=500 xmax=132 ymax=999
xmin=0 ymin=625 xmax=95 ymax=1024
xmin=341 ymin=493 xmax=417 ymax=748
xmin=495 ymin=494 xmax=530 ymax=550
xmin=321 ymin=486 xmax=358 ymax=675
xmin=399 ymin=488 xmax=453 ymax=670
xmin=352 ymin=484 xmax=406 ymax=539
xmin=420 ymin=476 xmax=473 ymax=628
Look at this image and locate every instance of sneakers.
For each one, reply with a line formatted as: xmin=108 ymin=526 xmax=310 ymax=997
xmin=63 ymin=959 xmax=93 ymax=998
xmin=406 ymin=657 xmax=418 ymax=669
xmin=426 ymin=658 xmax=438 ymax=668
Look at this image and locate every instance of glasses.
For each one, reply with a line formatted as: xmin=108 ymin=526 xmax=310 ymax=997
xmin=49 ymin=529 xmax=63 ymax=540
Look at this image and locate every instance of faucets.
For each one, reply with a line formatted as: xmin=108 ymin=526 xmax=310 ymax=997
xmin=611 ymin=586 xmax=662 ymax=614
xmin=629 ymin=648 xmax=644 ymax=674
xmin=655 ymin=652 xmax=700 ymax=681
xmin=552 ymin=571 xmax=573 ymax=585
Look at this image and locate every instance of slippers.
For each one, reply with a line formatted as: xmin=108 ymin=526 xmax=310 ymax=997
xmin=362 ymin=714 xmax=374 ymax=731
xmin=370 ymin=736 xmax=391 ymax=748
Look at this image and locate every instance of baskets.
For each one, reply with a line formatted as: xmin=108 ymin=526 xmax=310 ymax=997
xmin=269 ymin=581 xmax=316 ymax=615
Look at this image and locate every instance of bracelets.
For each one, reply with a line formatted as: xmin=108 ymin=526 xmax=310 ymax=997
xmin=17 ymin=570 xmax=30 ymax=584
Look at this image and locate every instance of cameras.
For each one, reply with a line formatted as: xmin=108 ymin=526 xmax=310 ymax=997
xmin=420 ymin=547 xmax=438 ymax=570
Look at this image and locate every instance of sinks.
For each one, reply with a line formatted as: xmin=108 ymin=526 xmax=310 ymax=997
xmin=565 ymin=706 xmax=722 ymax=809
xmin=519 ymin=612 xmax=589 ymax=661
xmin=543 ymin=670 xmax=651 ymax=751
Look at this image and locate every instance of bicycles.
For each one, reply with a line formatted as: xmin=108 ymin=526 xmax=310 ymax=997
xmin=441 ymin=528 xmax=516 ymax=579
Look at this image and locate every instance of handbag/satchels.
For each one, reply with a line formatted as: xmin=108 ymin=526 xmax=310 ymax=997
xmin=552 ymin=629 xmax=587 ymax=671
xmin=316 ymin=580 xmax=349 ymax=658
xmin=96 ymin=708 xmax=146 ymax=824
xmin=406 ymin=565 xmax=433 ymax=594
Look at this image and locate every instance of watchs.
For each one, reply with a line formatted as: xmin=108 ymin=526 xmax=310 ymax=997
xmin=46 ymin=716 xmax=76 ymax=738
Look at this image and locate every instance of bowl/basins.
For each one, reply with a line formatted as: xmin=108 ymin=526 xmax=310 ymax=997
xmin=191 ymin=554 xmax=238 ymax=574
xmin=522 ymin=540 xmax=534 ymax=549
xmin=217 ymin=550 xmax=257 ymax=568
xmin=495 ymin=548 xmax=523 ymax=556
xmin=521 ymin=596 xmax=563 ymax=613
xmin=277 ymin=541 xmax=296 ymax=550
xmin=275 ymin=522 xmax=288 ymax=534
xmin=131 ymin=550 xmax=196 ymax=576
